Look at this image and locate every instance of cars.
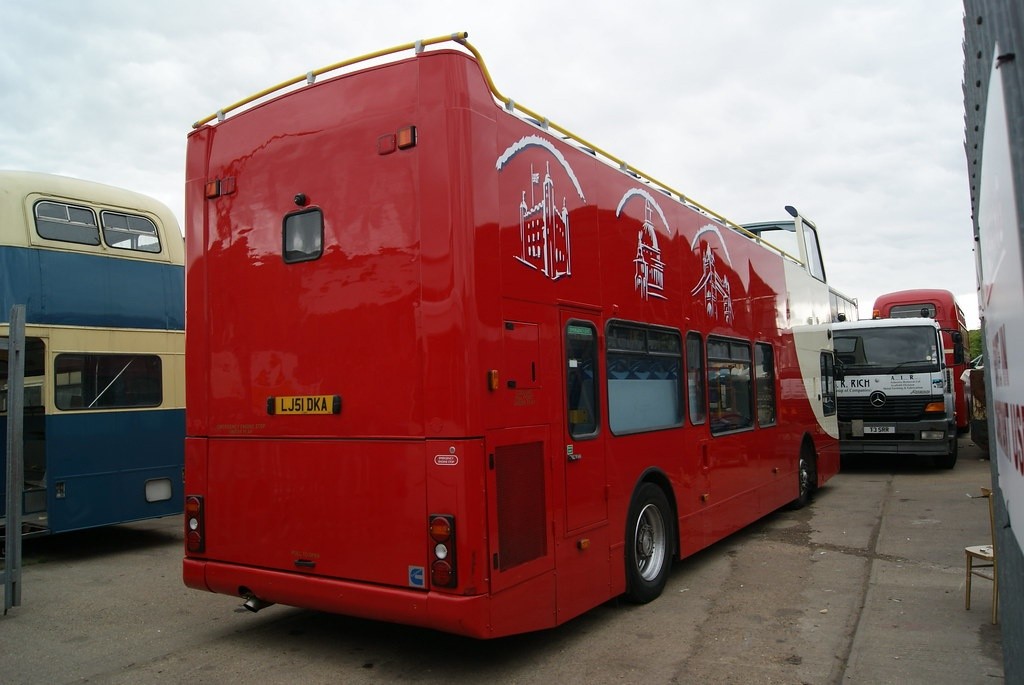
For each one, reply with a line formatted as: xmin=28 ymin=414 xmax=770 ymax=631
xmin=968 ymin=355 xmax=983 ymax=371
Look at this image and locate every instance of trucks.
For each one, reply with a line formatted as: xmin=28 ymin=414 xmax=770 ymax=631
xmin=827 ymin=317 xmax=964 ymax=472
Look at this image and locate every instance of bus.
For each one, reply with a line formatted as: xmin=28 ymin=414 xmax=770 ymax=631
xmin=177 ymin=34 xmax=842 ymax=642
xmin=1 ymin=167 xmax=190 ymax=547
xmin=873 ymin=289 xmax=970 ymax=435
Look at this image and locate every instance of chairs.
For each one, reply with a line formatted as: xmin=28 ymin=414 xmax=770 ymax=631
xmin=964 ymin=492 xmax=1001 ymax=625
